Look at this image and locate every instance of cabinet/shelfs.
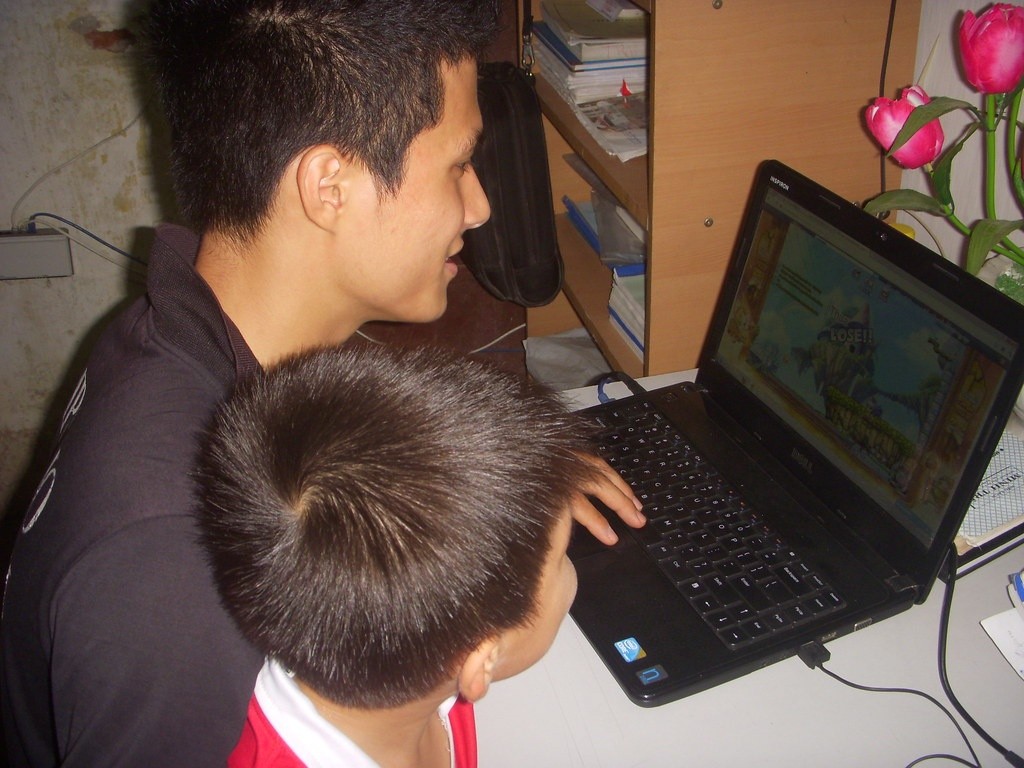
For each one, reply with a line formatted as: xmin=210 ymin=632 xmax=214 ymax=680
xmin=518 ymin=0 xmax=922 ymax=376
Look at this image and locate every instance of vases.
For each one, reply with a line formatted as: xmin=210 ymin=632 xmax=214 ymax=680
xmin=976 ymin=247 xmax=1024 ymax=420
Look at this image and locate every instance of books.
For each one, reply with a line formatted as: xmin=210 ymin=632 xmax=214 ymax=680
xmin=940 ymin=381 xmax=1022 ymax=680
xmin=528 ymin=1 xmax=647 ymax=361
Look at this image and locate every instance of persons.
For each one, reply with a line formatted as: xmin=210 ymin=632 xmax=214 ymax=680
xmin=189 ymin=338 xmax=579 ymax=768
xmin=1 ymin=1 xmax=646 ymax=767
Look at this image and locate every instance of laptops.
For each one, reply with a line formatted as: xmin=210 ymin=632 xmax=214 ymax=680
xmin=562 ymin=160 xmax=1024 ymax=707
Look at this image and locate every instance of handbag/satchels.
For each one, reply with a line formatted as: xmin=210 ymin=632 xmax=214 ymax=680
xmin=459 ymin=61 xmax=564 ymax=307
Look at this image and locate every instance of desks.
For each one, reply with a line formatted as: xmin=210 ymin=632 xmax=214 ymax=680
xmin=474 ymin=368 xmax=1024 ymax=768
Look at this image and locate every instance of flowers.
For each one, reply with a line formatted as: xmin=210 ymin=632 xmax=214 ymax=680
xmin=863 ymin=0 xmax=1024 ymax=276
